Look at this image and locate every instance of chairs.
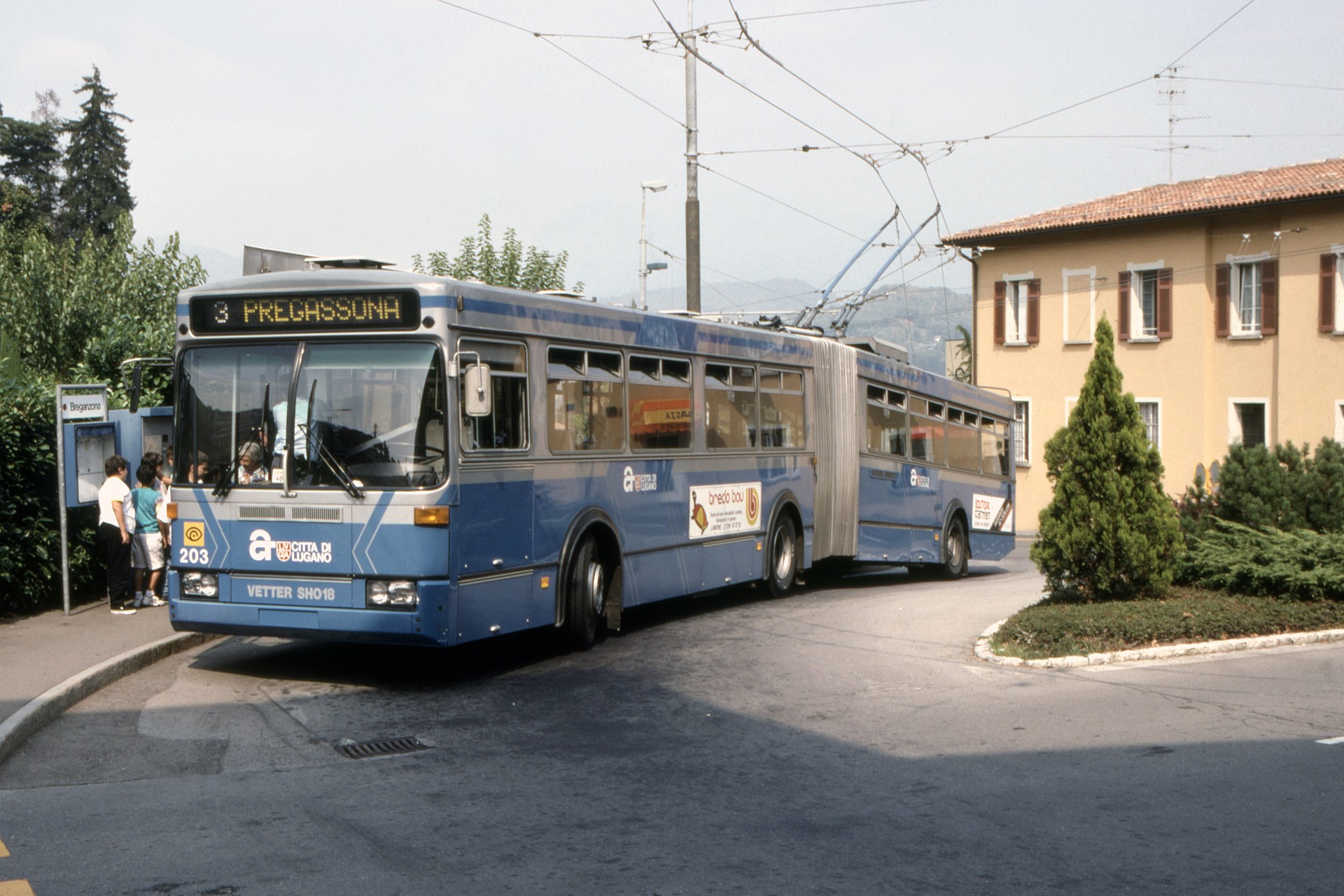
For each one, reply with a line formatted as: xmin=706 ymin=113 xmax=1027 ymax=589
xmin=425 ymin=417 xmax=474 ymax=481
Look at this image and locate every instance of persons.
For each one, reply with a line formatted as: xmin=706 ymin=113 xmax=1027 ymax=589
xmin=128 ymin=444 xmax=173 ymax=607
xmin=98 ymin=456 xmax=137 ymax=615
xmin=157 ymin=450 xmax=209 ymax=547
xmin=258 ymin=422 xmax=268 ymax=451
xmin=236 ymin=442 xmax=268 ymax=485
xmin=271 ymin=373 xmax=343 ymax=470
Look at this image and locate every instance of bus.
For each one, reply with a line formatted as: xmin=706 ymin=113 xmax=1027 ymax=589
xmin=118 ymin=201 xmax=1018 ymax=658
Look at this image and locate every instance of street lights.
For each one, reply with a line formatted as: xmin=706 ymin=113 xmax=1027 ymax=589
xmin=637 ymin=180 xmax=668 ymax=310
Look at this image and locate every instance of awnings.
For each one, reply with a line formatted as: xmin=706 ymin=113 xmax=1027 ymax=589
xmin=628 ymin=399 xmax=692 ymax=437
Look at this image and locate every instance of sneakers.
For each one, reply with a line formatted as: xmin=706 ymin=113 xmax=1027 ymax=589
xmin=110 ymin=605 xmax=137 ymax=614
xmin=142 ymin=596 xmax=165 ymax=607
xmin=133 ymin=597 xmax=143 ymax=607
